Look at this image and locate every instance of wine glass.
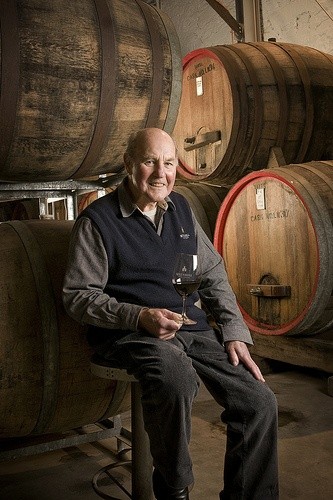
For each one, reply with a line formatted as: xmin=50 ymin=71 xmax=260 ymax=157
xmin=172 ymin=253 xmax=202 ymax=326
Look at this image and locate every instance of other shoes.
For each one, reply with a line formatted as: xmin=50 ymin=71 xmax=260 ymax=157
xmin=153 ymin=485 xmax=189 ymax=500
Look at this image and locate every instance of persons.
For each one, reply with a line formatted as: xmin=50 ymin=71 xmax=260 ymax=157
xmin=61 ymin=127 xmax=280 ymax=500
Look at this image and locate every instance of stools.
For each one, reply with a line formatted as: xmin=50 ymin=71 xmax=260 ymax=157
xmin=89 ymin=352 xmax=156 ymax=500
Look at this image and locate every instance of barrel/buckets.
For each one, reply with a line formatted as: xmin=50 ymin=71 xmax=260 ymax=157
xmin=214 ymin=161 xmax=333 ymax=338
xmin=79 ymin=183 xmax=230 ymax=244
xmin=171 ymin=42 xmax=333 ymax=187
xmin=0 ymin=222 xmax=131 ymax=434
xmin=0 ymin=0 xmax=182 ymax=179
